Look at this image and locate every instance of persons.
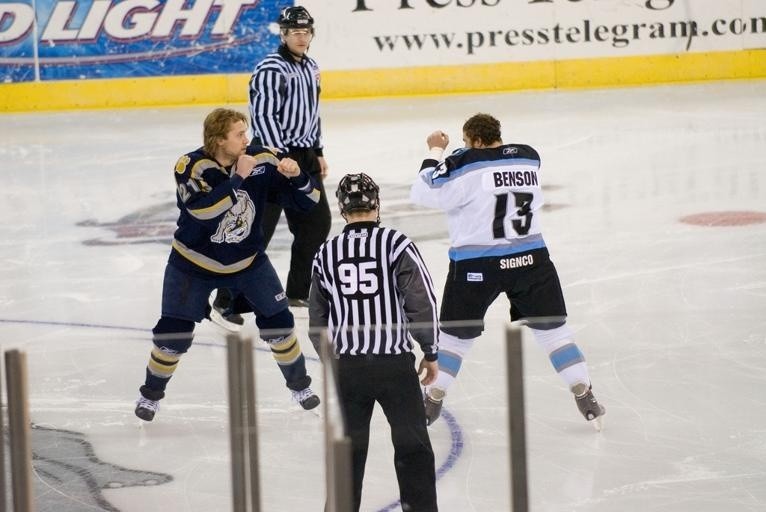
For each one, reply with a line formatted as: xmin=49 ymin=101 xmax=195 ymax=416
xmin=307 ymin=172 xmax=443 ymax=512
xmin=410 ymin=113 xmax=605 ymax=426
xmin=207 ymin=4 xmax=333 ymax=325
xmin=133 ymin=107 xmax=321 ymax=420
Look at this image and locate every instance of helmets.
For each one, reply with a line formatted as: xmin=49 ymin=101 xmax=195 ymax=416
xmin=278 ymin=5 xmax=314 ymax=30
xmin=336 ymin=172 xmax=379 ymax=213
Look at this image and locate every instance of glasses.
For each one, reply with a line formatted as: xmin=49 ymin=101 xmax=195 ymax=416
xmin=289 ymin=28 xmax=311 ymax=37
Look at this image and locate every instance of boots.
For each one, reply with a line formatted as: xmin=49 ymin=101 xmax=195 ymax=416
xmin=288 ymin=297 xmax=310 ymax=308
xmin=135 ymin=385 xmax=165 ymax=422
xmin=286 ymin=375 xmax=320 ymax=410
xmin=423 ymin=387 xmax=444 ymax=427
xmin=574 ymin=383 xmax=605 ymax=421
xmin=213 ymin=298 xmax=244 ymax=326
